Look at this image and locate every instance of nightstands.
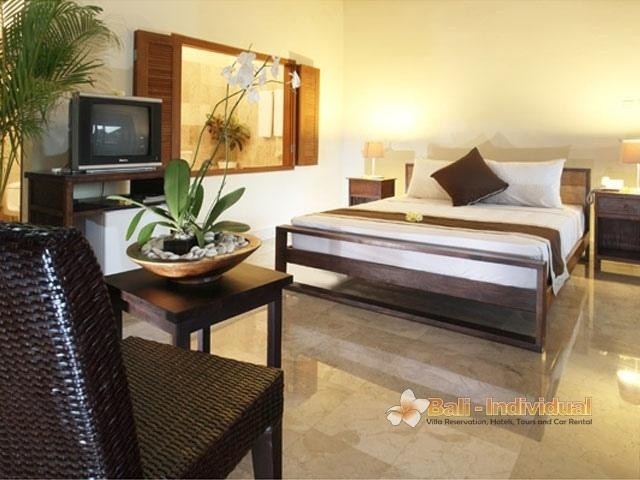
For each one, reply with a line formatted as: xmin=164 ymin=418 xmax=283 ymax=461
xmin=588 ymin=189 xmax=640 ymax=277
xmin=348 ymin=177 xmax=395 ymax=206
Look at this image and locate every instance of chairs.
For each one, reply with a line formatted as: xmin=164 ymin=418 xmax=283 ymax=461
xmin=1 ymin=219 xmax=284 ymax=479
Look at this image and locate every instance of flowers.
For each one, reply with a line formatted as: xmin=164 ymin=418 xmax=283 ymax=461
xmin=107 ymin=44 xmax=301 ymax=247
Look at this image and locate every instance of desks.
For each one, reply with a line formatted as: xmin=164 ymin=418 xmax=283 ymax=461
xmin=105 ymin=261 xmax=292 ymax=369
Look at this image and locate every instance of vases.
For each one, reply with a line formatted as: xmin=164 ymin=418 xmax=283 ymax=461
xmin=127 ymin=231 xmax=261 ymax=284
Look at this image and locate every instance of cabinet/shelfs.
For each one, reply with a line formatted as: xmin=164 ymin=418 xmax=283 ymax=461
xmin=24 ymin=164 xmax=172 ymax=227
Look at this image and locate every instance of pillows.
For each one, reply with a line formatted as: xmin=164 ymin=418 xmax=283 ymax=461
xmin=407 ymin=155 xmax=457 ymax=200
xmin=430 ymin=146 xmax=508 ymax=206
xmin=481 ymin=158 xmax=565 ymax=209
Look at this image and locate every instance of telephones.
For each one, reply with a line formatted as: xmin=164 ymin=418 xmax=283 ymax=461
xmin=585 ymin=176 xmax=624 ymax=205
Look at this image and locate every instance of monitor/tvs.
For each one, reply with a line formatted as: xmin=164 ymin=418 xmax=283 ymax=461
xmin=68 ymin=92 xmax=163 ymax=173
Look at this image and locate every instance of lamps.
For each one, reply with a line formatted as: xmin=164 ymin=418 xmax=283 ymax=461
xmin=363 ymin=141 xmax=384 ymax=178
xmin=621 ymin=139 xmax=640 ymax=193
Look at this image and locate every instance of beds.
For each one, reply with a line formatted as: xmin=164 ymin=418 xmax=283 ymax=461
xmin=274 ymin=165 xmax=592 ymax=352
